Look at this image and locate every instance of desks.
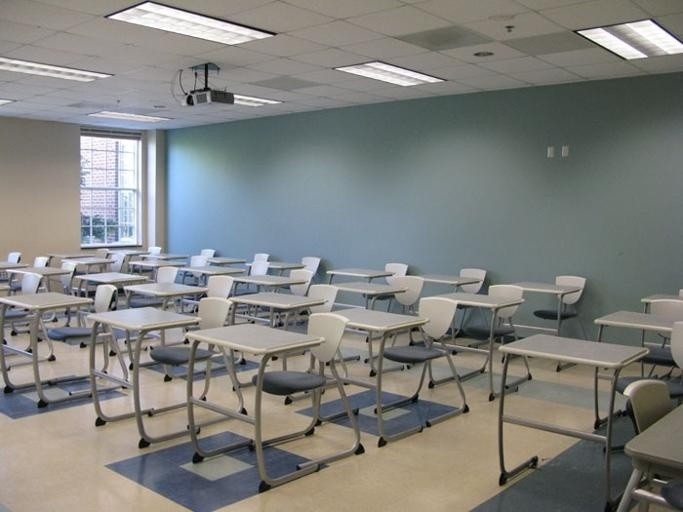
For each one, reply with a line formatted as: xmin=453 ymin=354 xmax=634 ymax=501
xmin=0 ymin=246 xmax=584 ymax=495
xmin=611 ymin=400 xmax=682 ymax=512
xmin=588 ymin=301 xmax=682 ymax=431
xmin=638 ymin=293 xmax=682 ymax=352
xmin=492 ymin=329 xmax=650 ymax=511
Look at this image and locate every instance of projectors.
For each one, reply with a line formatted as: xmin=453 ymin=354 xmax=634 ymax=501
xmin=179 ymin=89 xmax=234 ymax=108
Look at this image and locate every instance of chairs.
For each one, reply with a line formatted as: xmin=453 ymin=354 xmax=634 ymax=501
xmin=638 ymin=298 xmax=678 ymax=379
xmin=668 ymin=318 xmax=681 ymax=371
xmin=623 ymin=371 xmax=670 ymax=512
xmin=677 ymin=288 xmax=682 ymax=298
